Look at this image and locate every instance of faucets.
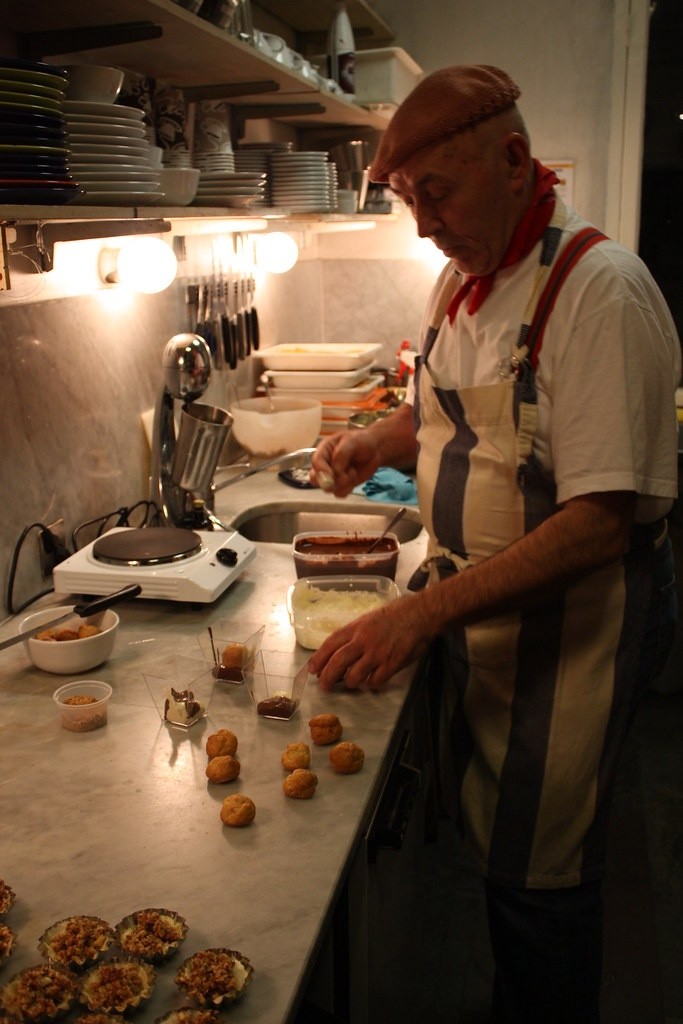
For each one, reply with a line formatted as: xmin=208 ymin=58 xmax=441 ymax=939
xmin=204 ymin=448 xmax=316 ymax=514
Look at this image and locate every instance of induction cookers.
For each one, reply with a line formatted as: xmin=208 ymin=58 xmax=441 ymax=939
xmin=52 ymin=527 xmax=257 ymax=603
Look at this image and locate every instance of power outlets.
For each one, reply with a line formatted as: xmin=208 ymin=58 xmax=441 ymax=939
xmin=37 ymin=518 xmax=69 ymax=577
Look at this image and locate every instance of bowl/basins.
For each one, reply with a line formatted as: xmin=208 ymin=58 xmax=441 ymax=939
xmin=292 ymin=530 xmax=400 ymax=583
xmin=336 ymin=190 xmax=358 ymax=214
xmin=230 ymin=396 xmax=323 ymax=457
xmin=347 ymin=408 xmax=396 ymax=429
xmin=148 ymin=145 xmax=201 ymax=206
xmin=20 ymin=605 xmax=120 ymax=675
xmin=287 ymin=574 xmax=403 ymax=650
xmin=53 ymin=680 xmax=113 ymax=732
xmin=60 ymin=64 xmax=126 ymax=103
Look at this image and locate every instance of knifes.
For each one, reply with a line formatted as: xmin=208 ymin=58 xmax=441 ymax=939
xmin=197 ymin=233 xmax=260 ymax=372
xmin=0 ymin=583 xmax=142 ymax=650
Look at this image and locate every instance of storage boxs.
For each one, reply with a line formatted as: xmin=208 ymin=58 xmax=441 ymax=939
xmin=307 ymin=46 xmax=424 ymax=112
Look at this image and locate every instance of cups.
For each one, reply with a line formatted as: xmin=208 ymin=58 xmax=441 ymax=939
xmin=253 ymin=28 xmax=342 ymax=96
xmin=120 ymin=70 xmax=238 ymax=171
xmin=141 ymin=652 xmax=220 ymax=727
xmin=197 ymin=617 xmax=266 ymax=684
xmin=240 ymin=648 xmax=312 ymax=720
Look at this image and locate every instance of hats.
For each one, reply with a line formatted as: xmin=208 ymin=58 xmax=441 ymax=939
xmin=368 ymin=64 xmax=521 ymax=183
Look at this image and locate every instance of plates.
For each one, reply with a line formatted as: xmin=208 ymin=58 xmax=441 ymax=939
xmin=253 ymin=342 xmax=391 ymax=439
xmin=65 ymin=99 xmax=167 ymax=206
xmin=278 ymin=470 xmax=315 ymax=489
xmin=0 ymin=58 xmax=84 ymax=204
xmin=200 ymin=140 xmax=340 ymax=213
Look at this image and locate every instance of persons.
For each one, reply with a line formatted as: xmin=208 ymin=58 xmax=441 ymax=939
xmin=314 ymin=65 xmax=683 ymax=1024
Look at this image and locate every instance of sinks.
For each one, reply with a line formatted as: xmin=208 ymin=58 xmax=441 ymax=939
xmin=229 ymin=502 xmax=421 ymax=546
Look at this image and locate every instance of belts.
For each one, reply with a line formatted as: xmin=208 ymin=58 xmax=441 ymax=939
xmin=623 ymin=516 xmax=669 ymax=565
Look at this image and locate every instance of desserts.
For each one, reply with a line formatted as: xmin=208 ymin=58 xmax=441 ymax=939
xmin=0 ymin=877 xmax=256 ymax=1024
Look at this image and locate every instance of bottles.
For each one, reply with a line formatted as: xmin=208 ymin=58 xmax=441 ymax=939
xmin=396 ymin=340 xmax=417 ymax=405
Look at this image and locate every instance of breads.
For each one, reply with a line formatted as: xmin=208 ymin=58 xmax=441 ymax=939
xmin=34 ymin=624 xmax=99 ymax=642
xmin=222 ymin=643 xmax=250 ymax=670
xmin=204 ymin=713 xmax=364 ymax=827
